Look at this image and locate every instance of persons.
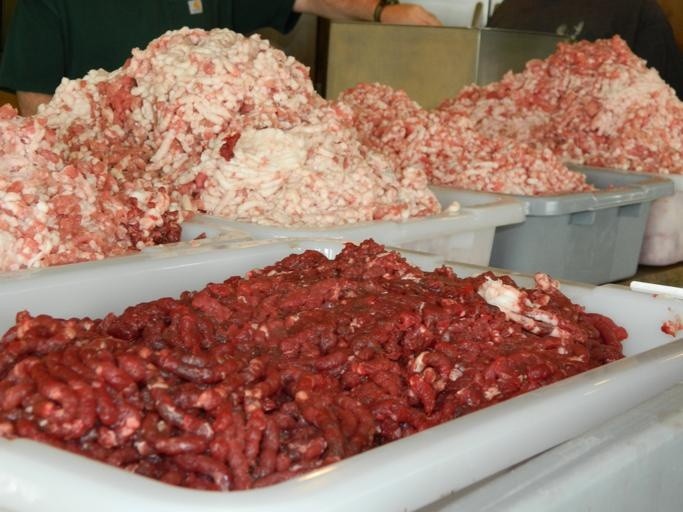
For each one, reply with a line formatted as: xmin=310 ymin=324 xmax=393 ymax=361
xmin=0 ymin=0 xmax=443 ymax=120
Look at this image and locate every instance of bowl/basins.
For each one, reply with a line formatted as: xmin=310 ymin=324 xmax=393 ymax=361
xmin=637 ymin=168 xmax=683 ymax=266
xmin=187 ymin=185 xmax=528 ymax=272
xmin=427 ymin=165 xmax=676 ymax=287
xmin=3 ymin=217 xmax=252 ymax=291
xmin=0 ymin=234 xmax=683 ymax=512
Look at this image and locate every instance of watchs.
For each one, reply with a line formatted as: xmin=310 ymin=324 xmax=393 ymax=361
xmin=372 ymin=1 xmax=402 ymax=26
